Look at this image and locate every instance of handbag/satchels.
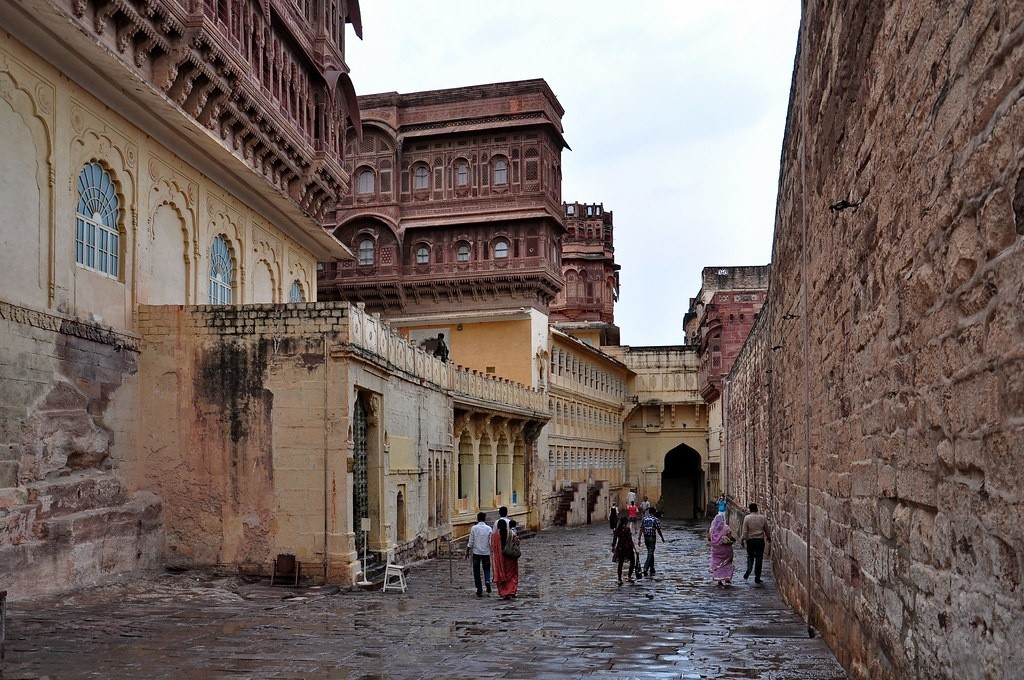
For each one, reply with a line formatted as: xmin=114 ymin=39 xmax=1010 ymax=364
xmin=503 ymin=531 xmax=521 ymax=558
xmin=635 ymin=553 xmax=643 ymax=579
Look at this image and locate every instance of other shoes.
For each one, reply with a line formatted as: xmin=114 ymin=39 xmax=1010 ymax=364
xmin=643 ymin=571 xmax=648 ymax=576
xmin=755 ymin=579 xmax=764 ymax=583
xmin=617 ymin=580 xmax=623 ymax=585
xmin=484 ymin=581 xmax=492 ymax=593
xmin=650 ymin=569 xmax=656 ymax=573
xmin=743 ymin=574 xmax=748 ymax=579
xmin=626 ymin=577 xmax=634 ymax=583
xmin=476 ymin=591 xmax=482 ymax=597
xmin=726 ymin=580 xmax=731 ymax=583
xmin=718 ymin=582 xmax=724 ymax=586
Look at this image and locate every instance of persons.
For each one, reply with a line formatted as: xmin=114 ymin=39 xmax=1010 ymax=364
xmin=741 ymin=503 xmax=771 ymax=584
xmin=608 ymin=502 xmax=619 ymax=533
xmin=465 ymin=512 xmax=492 ymax=597
xmin=642 ymin=496 xmax=650 ymax=519
xmin=656 ymin=496 xmax=665 ymax=517
xmin=637 ymin=507 xmax=665 ymax=575
xmin=509 ymin=520 xmax=517 ymax=535
xmin=492 ymin=506 xmax=510 ymax=583
xmin=491 ymin=519 xmax=520 ymax=599
xmin=627 ymin=501 xmax=639 ymax=534
xmin=434 ymin=333 xmax=449 ymax=363
xmin=708 ymin=514 xmax=736 ymax=584
xmin=716 ymin=494 xmax=726 ymax=521
xmin=611 ymin=517 xmax=639 ymax=584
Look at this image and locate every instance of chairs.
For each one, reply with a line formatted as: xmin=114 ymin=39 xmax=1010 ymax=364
xmin=382 ymin=550 xmax=408 ymax=594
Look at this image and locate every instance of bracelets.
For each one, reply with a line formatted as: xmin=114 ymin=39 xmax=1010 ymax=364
xmin=638 ymin=537 xmax=641 ymax=539
